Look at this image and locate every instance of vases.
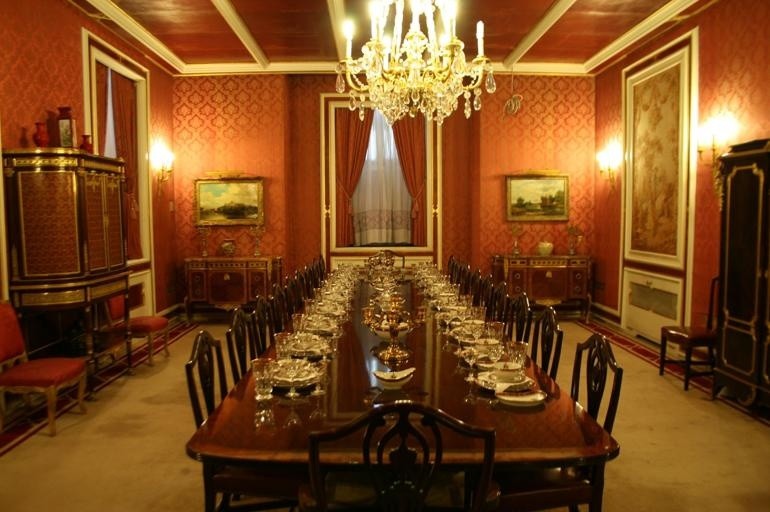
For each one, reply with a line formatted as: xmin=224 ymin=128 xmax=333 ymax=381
xmin=537 ymin=241 xmax=553 ymax=257
xmin=80 ymin=134 xmax=94 ymax=155
xmin=56 ymin=106 xmax=77 ymax=148
xmin=32 ymin=121 xmax=50 ymax=148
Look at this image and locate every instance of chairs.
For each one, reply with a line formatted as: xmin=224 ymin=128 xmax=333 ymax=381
xmin=104 ymin=294 xmax=170 ymax=366
xmin=659 ymin=277 xmax=719 ymax=391
xmin=0 ymin=300 xmax=88 ymax=436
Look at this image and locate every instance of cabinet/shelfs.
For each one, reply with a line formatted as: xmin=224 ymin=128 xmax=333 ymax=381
xmin=697 ymin=145 xmax=719 ymax=166
xmin=0 ymin=148 xmax=134 ymax=400
xmin=182 ymin=257 xmax=281 ymax=319
xmin=708 ymin=138 xmax=770 ymax=421
xmin=492 ymin=255 xmax=592 ymax=324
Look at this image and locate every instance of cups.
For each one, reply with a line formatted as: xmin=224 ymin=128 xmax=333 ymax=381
xmin=254 ymin=394 xmax=276 ymax=436
xmin=250 ymin=357 xmax=275 ymax=396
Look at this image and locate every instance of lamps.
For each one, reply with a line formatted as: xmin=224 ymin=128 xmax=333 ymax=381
xmin=599 ymin=167 xmax=617 ymax=188
xmin=335 ymin=0 xmax=496 ymax=128
xmin=159 ymin=168 xmax=173 ymax=182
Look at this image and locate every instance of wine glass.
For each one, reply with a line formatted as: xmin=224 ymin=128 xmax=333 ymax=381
xmin=285 ymin=260 xmax=506 ymax=405
xmin=283 ymin=400 xmax=327 ymax=428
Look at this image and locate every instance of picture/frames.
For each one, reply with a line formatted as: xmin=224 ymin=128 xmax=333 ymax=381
xmin=506 ymin=175 xmax=569 ymax=221
xmin=192 ymin=176 xmax=265 ymax=227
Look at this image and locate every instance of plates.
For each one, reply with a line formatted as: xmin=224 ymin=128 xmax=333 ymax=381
xmin=271 ymin=266 xmax=548 ymax=408
